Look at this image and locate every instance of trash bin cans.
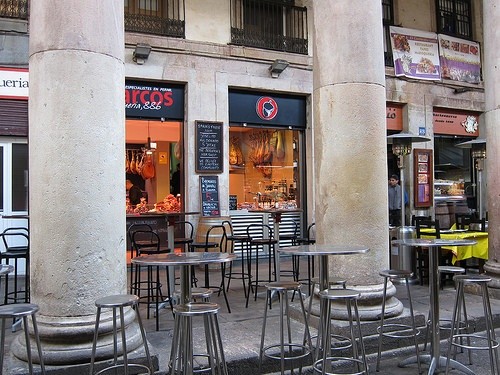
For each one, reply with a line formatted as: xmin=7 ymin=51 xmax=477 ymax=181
xmin=389 ymin=226 xmax=417 ymax=286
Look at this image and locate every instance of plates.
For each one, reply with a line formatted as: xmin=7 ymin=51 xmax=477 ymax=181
xmin=415 ymin=68 xmax=438 ymax=75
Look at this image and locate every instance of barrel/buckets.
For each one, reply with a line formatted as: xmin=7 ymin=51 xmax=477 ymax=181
xmin=196 ymin=216 xmax=234 ymax=271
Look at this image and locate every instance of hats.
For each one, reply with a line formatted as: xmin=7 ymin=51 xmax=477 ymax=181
xmin=391 ymin=174 xmax=400 ymax=180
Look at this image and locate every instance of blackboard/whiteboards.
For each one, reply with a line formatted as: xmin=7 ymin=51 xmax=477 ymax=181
xmin=229 ymin=194 xmax=237 ymax=210
xmin=195 ymin=121 xmax=223 ymax=173
xmin=199 ymin=175 xmax=220 ymax=218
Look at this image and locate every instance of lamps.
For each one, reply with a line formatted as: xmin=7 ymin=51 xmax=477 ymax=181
xmin=145 ymin=120 xmax=152 ymax=154
xmin=454 ymin=87 xmax=472 ymax=94
xmin=270 ymin=59 xmax=289 ymax=78
xmin=134 ymin=42 xmax=152 ymax=64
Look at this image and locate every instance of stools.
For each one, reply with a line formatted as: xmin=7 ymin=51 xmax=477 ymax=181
xmin=0 ymin=215 xmax=500 ymax=375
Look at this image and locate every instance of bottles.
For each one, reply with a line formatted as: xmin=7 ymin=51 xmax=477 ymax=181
xmin=275 ymin=200 xmax=278 ymax=209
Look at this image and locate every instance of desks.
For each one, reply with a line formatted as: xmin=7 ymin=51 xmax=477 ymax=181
xmin=391 ymin=239 xmax=478 ymax=375
xmin=277 ymin=245 xmax=371 ymax=375
xmin=141 ymin=211 xmax=201 ymax=317
xmin=248 ymin=208 xmax=303 ymax=303
xmin=131 ymin=251 xmax=236 ymax=375
xmin=418 ymin=229 xmax=489 ymax=286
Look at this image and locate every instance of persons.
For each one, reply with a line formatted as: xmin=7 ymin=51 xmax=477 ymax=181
xmin=388 ymin=175 xmax=408 ymax=226
xmin=126 ymin=180 xmax=143 ymax=208
xmin=171 ymin=163 xmax=180 ymax=195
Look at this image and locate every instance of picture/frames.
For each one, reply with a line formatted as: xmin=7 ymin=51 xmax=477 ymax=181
xmin=413 ymin=148 xmax=433 ymax=208
